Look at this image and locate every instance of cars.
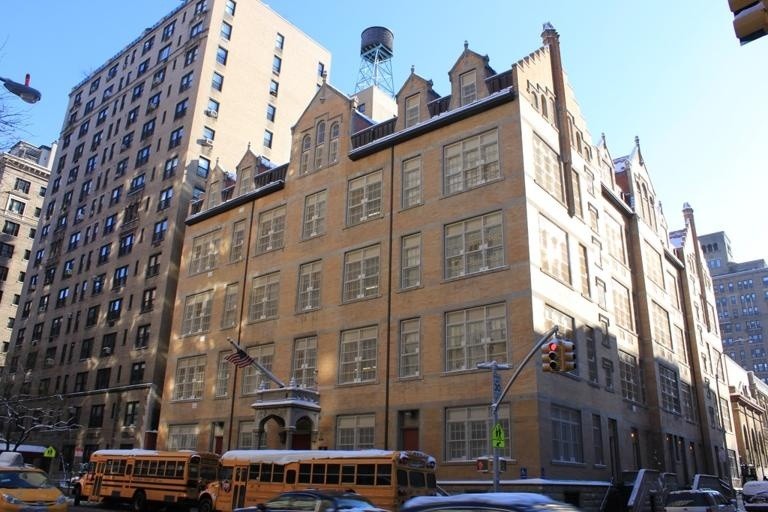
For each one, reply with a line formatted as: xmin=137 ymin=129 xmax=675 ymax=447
xmin=232 ymin=487 xmax=386 ymax=510
xmin=0 ymin=450 xmax=68 ymax=511
xmin=406 ymin=491 xmax=578 ymax=511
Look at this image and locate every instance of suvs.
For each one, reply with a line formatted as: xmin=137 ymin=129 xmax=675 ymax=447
xmin=665 ymin=488 xmax=737 ymax=512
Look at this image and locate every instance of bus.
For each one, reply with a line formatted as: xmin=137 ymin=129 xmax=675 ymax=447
xmin=200 ymin=447 xmax=439 ymax=512
xmin=69 ymin=447 xmax=223 ymax=510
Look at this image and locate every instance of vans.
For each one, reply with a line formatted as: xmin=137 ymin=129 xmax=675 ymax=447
xmin=741 ymin=480 xmax=768 ymax=510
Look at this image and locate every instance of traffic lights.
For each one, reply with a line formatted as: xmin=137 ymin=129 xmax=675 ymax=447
xmin=728 ymin=1 xmax=768 ymax=46
xmin=561 ymin=340 xmax=577 ymax=371
xmin=541 ymin=343 xmax=550 ymax=372
xmin=548 ymin=339 xmax=563 ymax=373
